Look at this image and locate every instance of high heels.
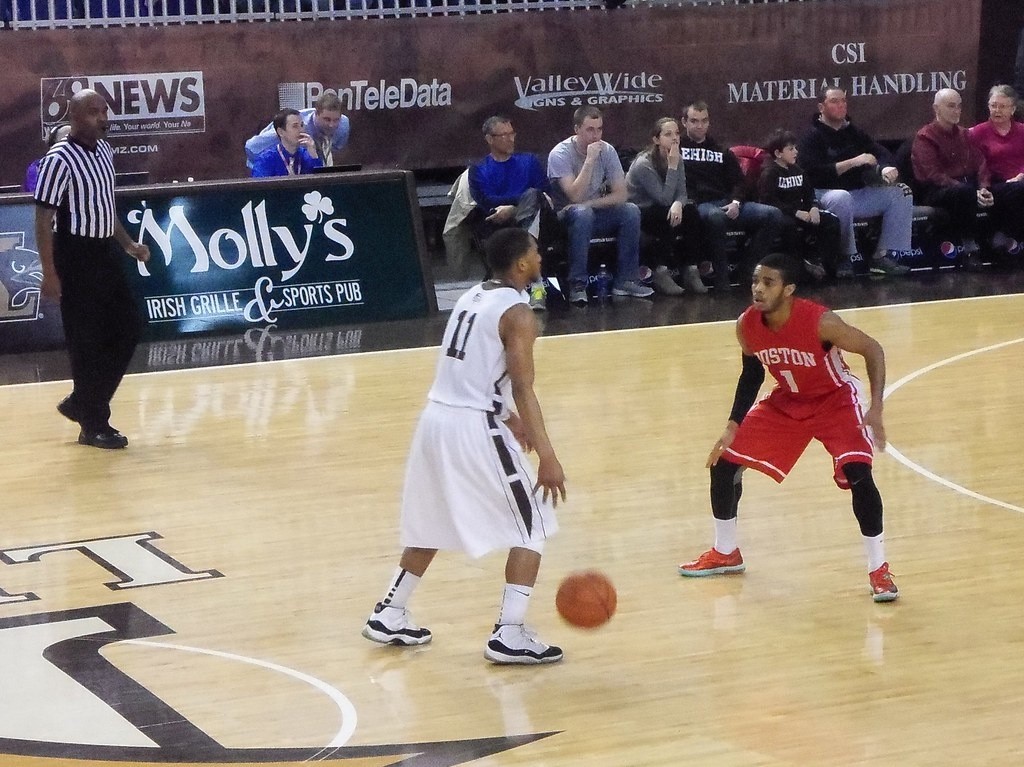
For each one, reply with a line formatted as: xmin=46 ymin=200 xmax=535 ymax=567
xmin=683 ymin=266 xmax=708 ymax=293
xmin=653 ymin=269 xmax=686 ymax=295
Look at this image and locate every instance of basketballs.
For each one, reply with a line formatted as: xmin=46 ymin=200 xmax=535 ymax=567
xmin=556 ymin=571 xmax=617 ymax=628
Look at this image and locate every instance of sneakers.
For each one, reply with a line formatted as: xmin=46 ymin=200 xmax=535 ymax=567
xmin=869 ymin=562 xmax=898 ymax=601
xmin=833 ymin=254 xmax=853 ymax=279
xmin=870 ymin=254 xmax=910 ymax=276
xmin=362 ymin=602 xmax=432 ymax=645
xmin=484 ymin=623 xmax=563 ymax=664
xmin=570 ymin=285 xmax=588 ymax=303
xmin=79 ymin=425 xmax=128 ymax=449
xmin=611 ymin=278 xmax=654 ymax=297
xmin=58 ymin=392 xmax=77 ymax=422
xmin=679 ymin=547 xmax=745 ymax=576
xmin=530 ymin=288 xmax=547 ymax=311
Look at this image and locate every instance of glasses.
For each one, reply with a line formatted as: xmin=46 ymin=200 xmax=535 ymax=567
xmin=987 ymin=103 xmax=1014 ymax=110
xmin=489 ymin=130 xmax=516 ymax=138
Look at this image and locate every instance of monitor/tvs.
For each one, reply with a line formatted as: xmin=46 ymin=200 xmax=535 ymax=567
xmin=312 ymin=164 xmax=362 ymax=174
xmin=116 ymin=172 xmax=150 ymax=186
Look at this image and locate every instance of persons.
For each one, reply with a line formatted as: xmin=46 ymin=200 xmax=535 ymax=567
xmin=32 ymin=89 xmax=150 ymax=449
xmin=468 ymin=116 xmax=552 ymax=311
xmin=797 ymin=84 xmax=913 ymax=275
xmin=678 ymin=99 xmax=785 ymax=291
xmin=245 ymin=93 xmax=350 ymax=180
xmin=756 ymin=127 xmax=845 ymax=285
xmin=26 ymin=124 xmax=73 ymax=192
xmin=362 ymin=227 xmax=564 ymax=665
xmin=623 ymin=117 xmax=709 ymax=295
xmin=910 ymin=80 xmax=1024 ymax=268
xmin=679 ymin=253 xmax=899 ymax=602
xmin=547 ymin=105 xmax=654 ymax=304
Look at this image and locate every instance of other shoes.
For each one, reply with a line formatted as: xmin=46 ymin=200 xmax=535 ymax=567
xmin=712 ymin=273 xmax=731 ymax=291
xmin=806 ymin=258 xmax=826 ymax=281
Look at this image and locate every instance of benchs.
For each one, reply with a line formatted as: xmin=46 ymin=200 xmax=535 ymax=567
xmin=442 ymin=139 xmax=994 ymax=299
